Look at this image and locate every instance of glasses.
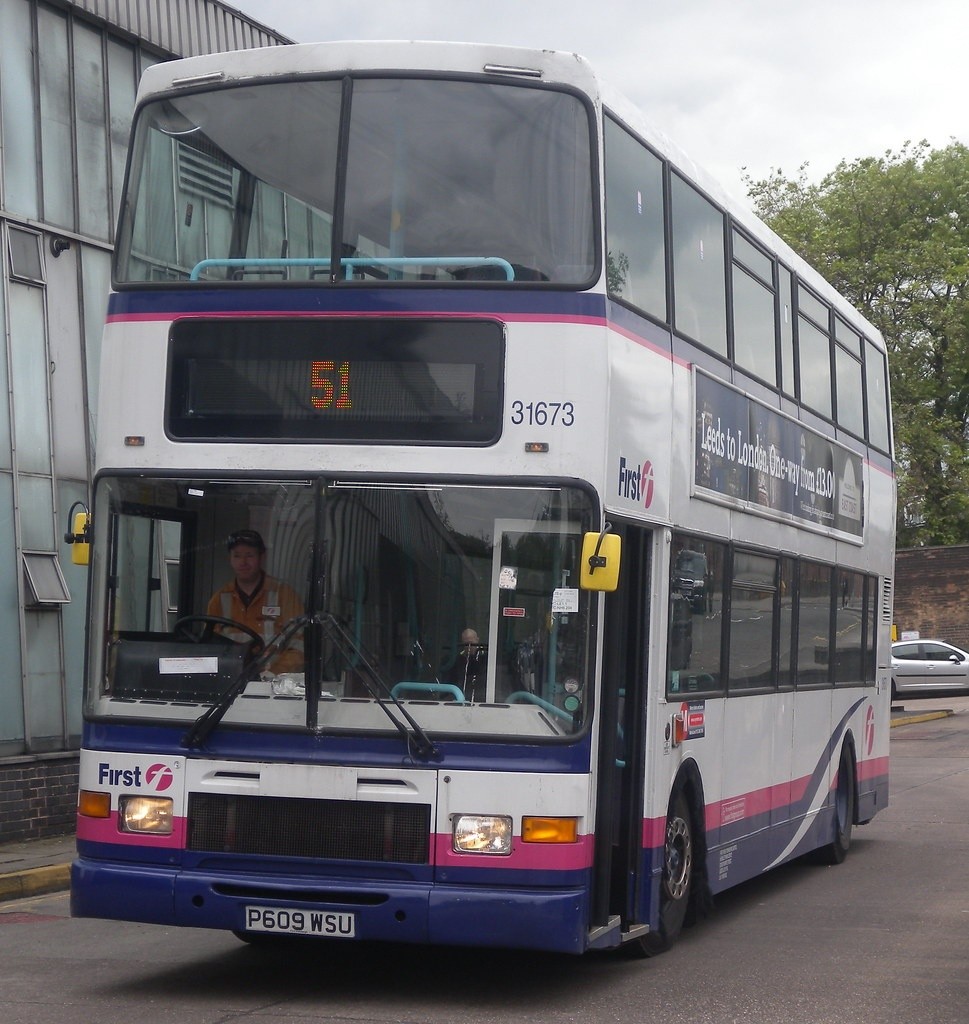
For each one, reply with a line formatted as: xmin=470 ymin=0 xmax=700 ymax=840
xmin=227 ymin=531 xmax=256 ymax=544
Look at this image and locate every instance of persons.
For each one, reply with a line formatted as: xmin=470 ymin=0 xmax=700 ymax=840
xmin=840 ymin=572 xmax=849 ymax=610
xmin=207 ymin=531 xmax=307 ymax=682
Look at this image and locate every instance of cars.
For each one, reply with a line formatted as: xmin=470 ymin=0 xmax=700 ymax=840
xmin=890 ymin=638 xmax=969 ymax=701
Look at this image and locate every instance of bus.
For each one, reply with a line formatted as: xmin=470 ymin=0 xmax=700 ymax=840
xmin=64 ymin=39 xmax=898 ymax=961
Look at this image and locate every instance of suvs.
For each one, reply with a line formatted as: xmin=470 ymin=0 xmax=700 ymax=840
xmin=671 ymin=548 xmax=710 ymax=613
